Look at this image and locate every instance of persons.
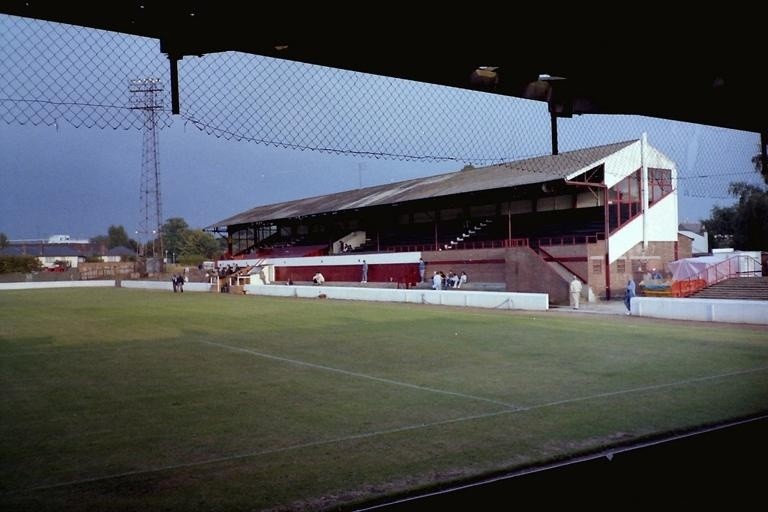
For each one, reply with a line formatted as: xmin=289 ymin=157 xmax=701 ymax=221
xmin=360 ymin=259 xmax=368 ymax=283
xmin=419 ymin=257 xmax=425 ymax=283
xmin=650 ymin=267 xmax=661 ymax=280
xmin=431 ymin=269 xmax=468 ymax=290
xmin=216 ymin=263 xmax=241 ymax=279
xmin=316 ymin=272 xmax=325 ymax=284
xmin=285 ymin=277 xmax=293 ymax=285
xmin=623 ymin=273 xmax=635 ymax=315
xmin=313 ymin=272 xmax=318 ymax=283
xmin=172 ymin=273 xmax=179 ymax=293
xmin=178 ymin=274 xmax=184 ymax=292
xmin=569 ymin=275 xmax=583 ymax=311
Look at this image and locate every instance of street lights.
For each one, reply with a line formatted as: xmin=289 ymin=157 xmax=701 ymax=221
xmin=129 ymin=78 xmax=165 ymax=256
